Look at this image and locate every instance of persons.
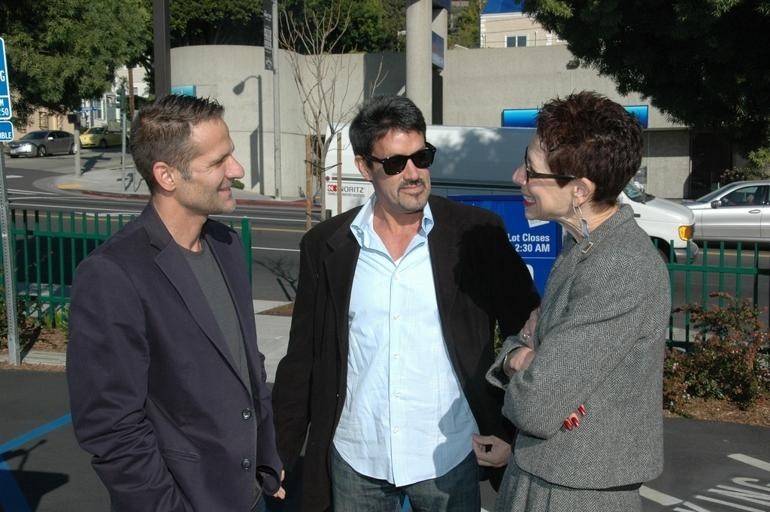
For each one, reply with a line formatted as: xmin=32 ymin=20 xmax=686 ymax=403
xmin=66 ymin=94 xmax=287 ymax=512
xmin=485 ymin=90 xmax=670 ymax=511
xmin=269 ymin=93 xmax=543 ymax=512
xmin=722 ymin=193 xmax=755 ymax=206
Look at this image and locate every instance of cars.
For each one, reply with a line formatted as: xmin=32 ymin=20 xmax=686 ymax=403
xmin=3 ymin=128 xmax=81 ymax=159
xmin=78 ymin=125 xmax=130 ymax=151
xmin=682 ymin=176 xmax=770 ymax=248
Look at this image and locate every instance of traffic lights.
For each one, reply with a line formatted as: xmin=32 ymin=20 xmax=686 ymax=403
xmin=116 ymin=87 xmax=125 ymax=111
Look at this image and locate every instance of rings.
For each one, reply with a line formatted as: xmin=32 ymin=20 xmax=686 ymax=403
xmin=521 ymin=334 xmax=530 ymax=340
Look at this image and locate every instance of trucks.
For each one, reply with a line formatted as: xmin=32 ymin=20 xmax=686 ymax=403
xmin=319 ymin=122 xmax=705 ymax=269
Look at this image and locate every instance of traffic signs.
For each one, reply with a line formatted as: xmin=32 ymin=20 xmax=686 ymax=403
xmin=0 ymin=36 xmax=13 ymax=121
xmin=0 ymin=121 xmax=14 ymax=144
xmin=79 ymin=107 xmax=97 ymax=113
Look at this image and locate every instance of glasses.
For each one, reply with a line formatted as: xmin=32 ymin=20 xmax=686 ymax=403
xmin=524 ymin=145 xmax=576 ymax=184
xmin=362 ymin=142 xmax=437 ymax=176
